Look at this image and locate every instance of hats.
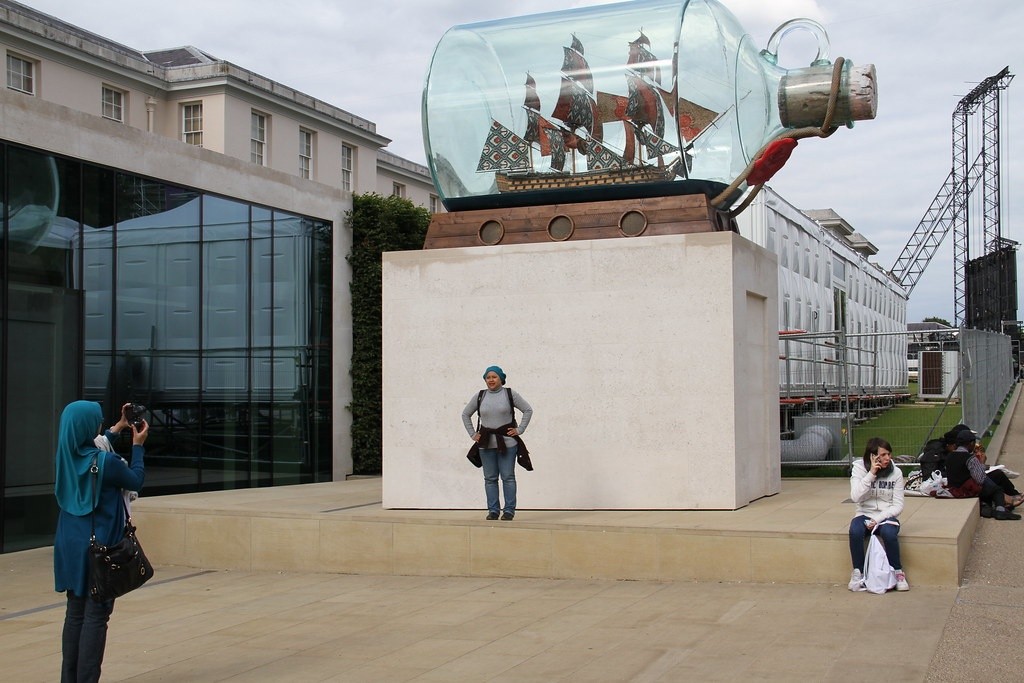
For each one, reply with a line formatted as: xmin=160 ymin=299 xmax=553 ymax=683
xmin=944 ymin=429 xmax=959 ymax=438
xmin=952 ymin=424 xmax=978 ymax=434
xmin=483 ymin=366 xmax=506 ymax=381
xmin=956 ymin=430 xmax=980 ymax=442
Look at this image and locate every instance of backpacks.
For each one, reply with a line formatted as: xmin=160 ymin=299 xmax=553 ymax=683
xmin=852 ymin=520 xmax=900 ymax=595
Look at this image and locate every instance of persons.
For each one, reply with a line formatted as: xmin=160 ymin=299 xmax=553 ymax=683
xmin=920 ymin=424 xmax=1024 ymax=520
xmin=53 ymin=400 xmax=154 ymax=683
xmin=462 ymin=366 xmax=533 ymax=521
xmin=848 ymin=437 xmax=909 ymax=591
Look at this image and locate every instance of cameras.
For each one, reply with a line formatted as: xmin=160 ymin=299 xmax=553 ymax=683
xmin=124 ymin=404 xmax=146 ymax=427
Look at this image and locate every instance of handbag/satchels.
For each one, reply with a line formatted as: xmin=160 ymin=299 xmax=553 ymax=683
xmin=904 ymin=470 xmax=923 ymax=490
xmin=83 ymin=523 xmax=154 ymax=603
xmin=915 ymin=470 xmax=955 ymax=498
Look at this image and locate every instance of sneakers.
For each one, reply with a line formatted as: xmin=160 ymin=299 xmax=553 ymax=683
xmin=895 ymin=569 xmax=910 ymax=590
xmin=847 ymin=568 xmax=863 ymax=591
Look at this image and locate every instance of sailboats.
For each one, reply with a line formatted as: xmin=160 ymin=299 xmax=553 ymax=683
xmin=476 ymin=26 xmax=753 ymax=193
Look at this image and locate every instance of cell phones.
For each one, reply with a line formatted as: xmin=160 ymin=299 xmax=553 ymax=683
xmin=872 ymin=454 xmax=881 ymax=464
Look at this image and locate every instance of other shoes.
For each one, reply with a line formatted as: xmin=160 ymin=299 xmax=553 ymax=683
xmin=980 ymin=501 xmax=993 ymax=518
xmin=486 ymin=512 xmax=499 ymax=520
xmin=501 ymin=512 xmax=514 ymax=520
xmin=995 ymin=508 xmax=1021 ymax=520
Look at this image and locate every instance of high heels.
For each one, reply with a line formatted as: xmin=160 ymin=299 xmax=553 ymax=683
xmin=1004 ymin=492 xmax=1024 ymax=512
